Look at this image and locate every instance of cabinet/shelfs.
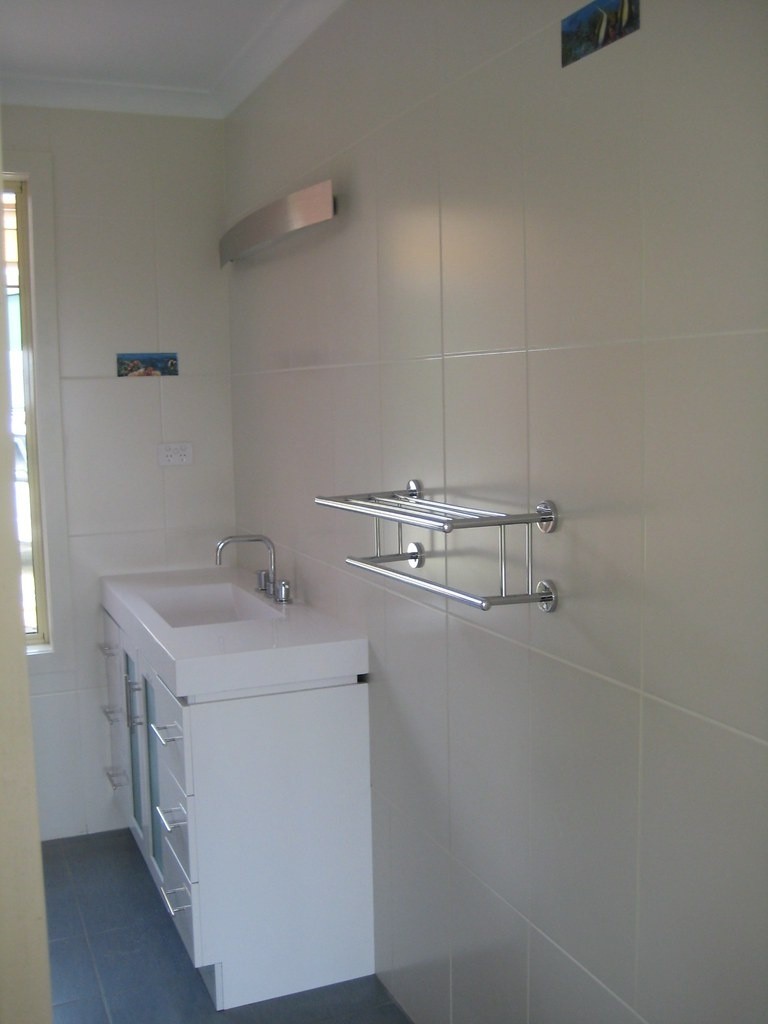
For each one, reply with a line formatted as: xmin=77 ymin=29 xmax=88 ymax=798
xmin=97 ymin=604 xmax=377 ymax=1012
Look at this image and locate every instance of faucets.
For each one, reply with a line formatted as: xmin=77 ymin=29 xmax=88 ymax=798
xmin=216 ymin=534 xmax=277 ymax=599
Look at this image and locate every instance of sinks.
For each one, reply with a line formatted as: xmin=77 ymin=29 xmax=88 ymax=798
xmin=138 ymin=581 xmax=288 ymax=629
